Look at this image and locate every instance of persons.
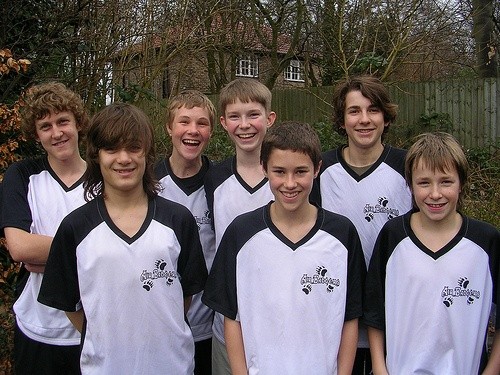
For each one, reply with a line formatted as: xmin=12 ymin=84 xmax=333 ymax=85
xmin=37 ymin=102 xmax=209 ymax=375
xmin=201 ymin=122 xmax=368 ymax=375
xmin=146 ymin=89 xmax=216 ymax=374
xmin=0 ymin=84 xmax=103 ymax=375
xmin=361 ymin=131 xmax=500 ymax=375
xmin=307 ymin=75 xmax=413 ymax=375
xmin=205 ymin=76 xmax=276 ymax=375
